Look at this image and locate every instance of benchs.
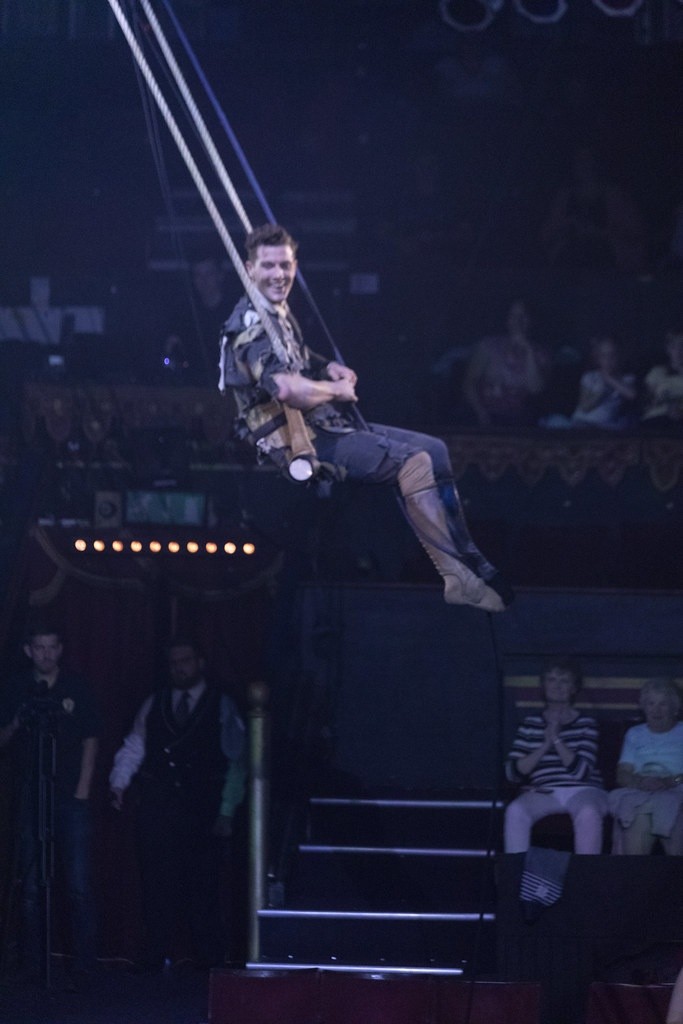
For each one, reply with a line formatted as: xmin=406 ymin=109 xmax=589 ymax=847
xmin=497 ymin=670 xmax=683 ymax=830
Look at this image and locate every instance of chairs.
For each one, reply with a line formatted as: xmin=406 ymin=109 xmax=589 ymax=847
xmin=207 ymin=968 xmax=543 ymax=1023
xmin=587 ymin=982 xmax=675 ymax=1024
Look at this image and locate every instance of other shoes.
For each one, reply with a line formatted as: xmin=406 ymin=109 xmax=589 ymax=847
xmin=125 ymin=953 xmax=166 ymax=977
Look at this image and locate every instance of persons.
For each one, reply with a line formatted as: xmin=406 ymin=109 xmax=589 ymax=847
xmin=219 ymin=222 xmax=504 ymax=609
xmin=1 ymin=258 xmax=683 ymax=988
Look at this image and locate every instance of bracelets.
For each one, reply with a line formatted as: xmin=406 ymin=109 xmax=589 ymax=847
xmin=554 ymin=738 xmax=561 ymax=746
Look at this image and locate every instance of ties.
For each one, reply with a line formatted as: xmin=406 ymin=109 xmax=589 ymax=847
xmin=175 ymin=693 xmax=192 ymax=730
